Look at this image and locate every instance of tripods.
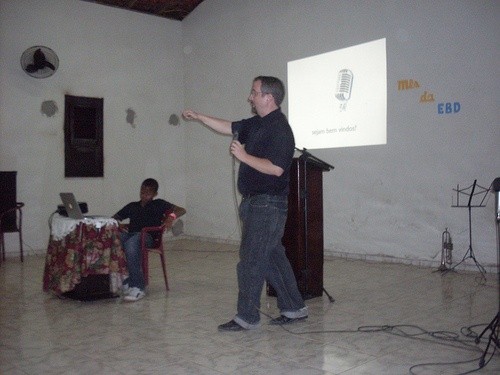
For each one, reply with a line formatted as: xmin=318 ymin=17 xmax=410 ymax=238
xmin=441 ymin=181 xmax=491 ymax=280
xmin=293 ymin=147 xmax=337 ymax=302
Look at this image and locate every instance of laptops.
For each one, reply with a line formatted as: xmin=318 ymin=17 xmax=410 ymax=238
xmin=59 ymin=192 xmax=97 ymax=219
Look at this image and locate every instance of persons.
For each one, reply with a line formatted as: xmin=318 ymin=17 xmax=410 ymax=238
xmin=182 ymin=75 xmax=309 ymax=330
xmin=110 ymin=178 xmax=187 ymax=301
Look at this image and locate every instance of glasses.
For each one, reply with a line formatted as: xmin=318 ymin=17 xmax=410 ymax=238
xmin=251 ymin=89 xmax=273 ymax=99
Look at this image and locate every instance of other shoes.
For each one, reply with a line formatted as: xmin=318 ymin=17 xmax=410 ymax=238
xmin=217 ymin=320 xmax=250 ymax=332
xmin=271 ymin=315 xmax=308 ymax=325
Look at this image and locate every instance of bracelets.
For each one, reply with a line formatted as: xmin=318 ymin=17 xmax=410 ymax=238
xmin=168 ymin=213 xmax=177 ymax=221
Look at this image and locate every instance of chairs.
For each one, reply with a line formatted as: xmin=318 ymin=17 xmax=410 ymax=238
xmin=0 ymin=202 xmax=25 ymax=264
xmin=120 ymin=210 xmax=170 ymax=293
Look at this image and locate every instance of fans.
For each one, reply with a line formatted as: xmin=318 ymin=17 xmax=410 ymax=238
xmin=20 ymin=44 xmax=60 ymax=80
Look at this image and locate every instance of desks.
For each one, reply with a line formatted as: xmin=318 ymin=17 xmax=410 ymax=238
xmin=43 ymin=213 xmax=121 ymax=301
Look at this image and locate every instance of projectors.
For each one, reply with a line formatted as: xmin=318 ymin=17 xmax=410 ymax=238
xmin=57 ymin=202 xmax=88 ymax=217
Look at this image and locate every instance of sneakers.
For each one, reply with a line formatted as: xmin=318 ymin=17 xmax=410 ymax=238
xmin=124 ymin=286 xmax=146 ymax=301
